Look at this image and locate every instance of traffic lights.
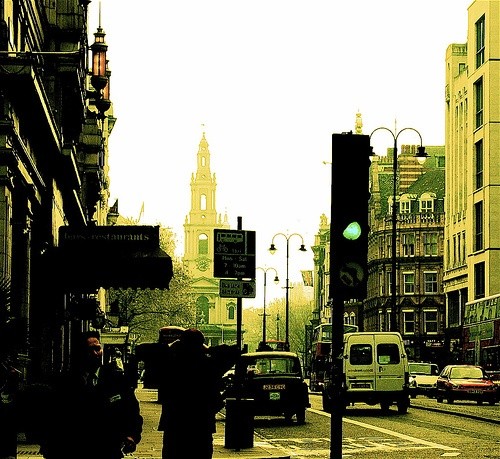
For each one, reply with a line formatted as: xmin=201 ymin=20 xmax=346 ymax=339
xmin=330 ymin=134 xmax=368 ymax=301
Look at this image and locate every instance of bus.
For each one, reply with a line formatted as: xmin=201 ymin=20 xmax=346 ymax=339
xmin=258 ymin=340 xmax=290 ymax=352
xmin=308 ymin=322 xmax=359 ymax=394
xmin=159 ymin=325 xmax=187 ymax=343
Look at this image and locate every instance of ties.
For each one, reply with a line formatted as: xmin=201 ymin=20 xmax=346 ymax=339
xmin=86 ymin=373 xmax=95 ymax=388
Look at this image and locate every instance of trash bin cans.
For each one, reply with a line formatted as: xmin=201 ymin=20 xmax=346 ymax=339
xmin=225 ymin=398 xmax=254 ymax=451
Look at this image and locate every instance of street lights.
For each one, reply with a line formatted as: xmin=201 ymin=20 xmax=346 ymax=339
xmin=255 ymin=266 xmax=280 ymax=350
xmin=368 ymin=127 xmax=431 ymax=334
xmin=268 ymin=232 xmax=307 ymax=349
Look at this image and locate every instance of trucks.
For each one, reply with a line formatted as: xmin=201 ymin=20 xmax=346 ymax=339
xmin=322 ymin=330 xmax=411 ymax=414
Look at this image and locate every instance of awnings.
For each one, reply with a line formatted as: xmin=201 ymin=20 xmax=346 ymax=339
xmin=52 ymin=225 xmax=173 ymax=292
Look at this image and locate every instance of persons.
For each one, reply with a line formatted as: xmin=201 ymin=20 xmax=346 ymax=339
xmin=33 ymin=328 xmax=229 ymax=459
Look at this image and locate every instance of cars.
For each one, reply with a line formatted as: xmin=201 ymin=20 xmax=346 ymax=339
xmin=241 ymin=350 xmax=311 ymax=424
xmin=405 ymin=361 xmax=442 ymax=399
xmin=437 ymin=365 xmax=495 ymax=404
